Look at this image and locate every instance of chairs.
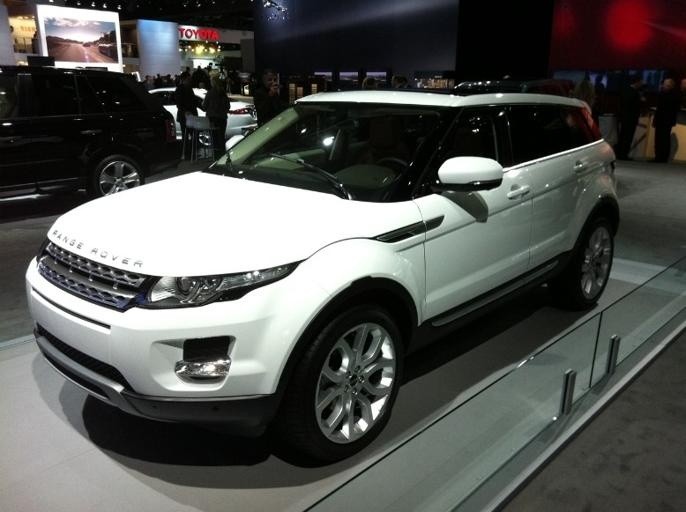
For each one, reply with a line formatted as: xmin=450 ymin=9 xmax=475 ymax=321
xmin=181 ymin=112 xmax=220 ymax=165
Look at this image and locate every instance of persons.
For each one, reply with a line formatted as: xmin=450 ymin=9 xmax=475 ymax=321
xmin=401 ymin=76 xmax=412 ymax=88
xmin=144 ymin=67 xmax=247 ymax=95
xmin=647 ymin=79 xmax=680 ymax=163
xmin=614 ymin=72 xmax=644 ymax=161
xmin=200 ymin=69 xmax=227 ymax=159
xmin=389 ymin=75 xmax=405 ymax=89
xmin=360 ymin=76 xmax=379 ymax=90
xmin=172 ymin=72 xmax=203 ymax=142
xmin=253 ymin=69 xmax=286 ymax=125
xmin=574 ymin=80 xmax=603 ymax=134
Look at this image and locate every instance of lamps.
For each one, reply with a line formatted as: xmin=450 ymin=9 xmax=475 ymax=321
xmin=77 ymin=2 xmax=121 ymax=11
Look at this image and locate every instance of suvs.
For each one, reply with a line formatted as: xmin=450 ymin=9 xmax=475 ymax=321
xmin=21 ymin=91 xmax=622 ymax=461
xmin=0 ymin=65 xmax=180 ymax=208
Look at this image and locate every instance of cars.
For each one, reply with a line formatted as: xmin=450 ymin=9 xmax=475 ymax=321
xmin=147 ymin=86 xmax=259 ymax=147
xmin=83 ymin=42 xmax=91 ymax=47
xmin=97 ymin=43 xmax=117 ymax=60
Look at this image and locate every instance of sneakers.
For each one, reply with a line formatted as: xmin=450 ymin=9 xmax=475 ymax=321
xmin=647 ymin=157 xmax=669 ymax=164
xmin=617 ymin=155 xmax=634 ymax=161
